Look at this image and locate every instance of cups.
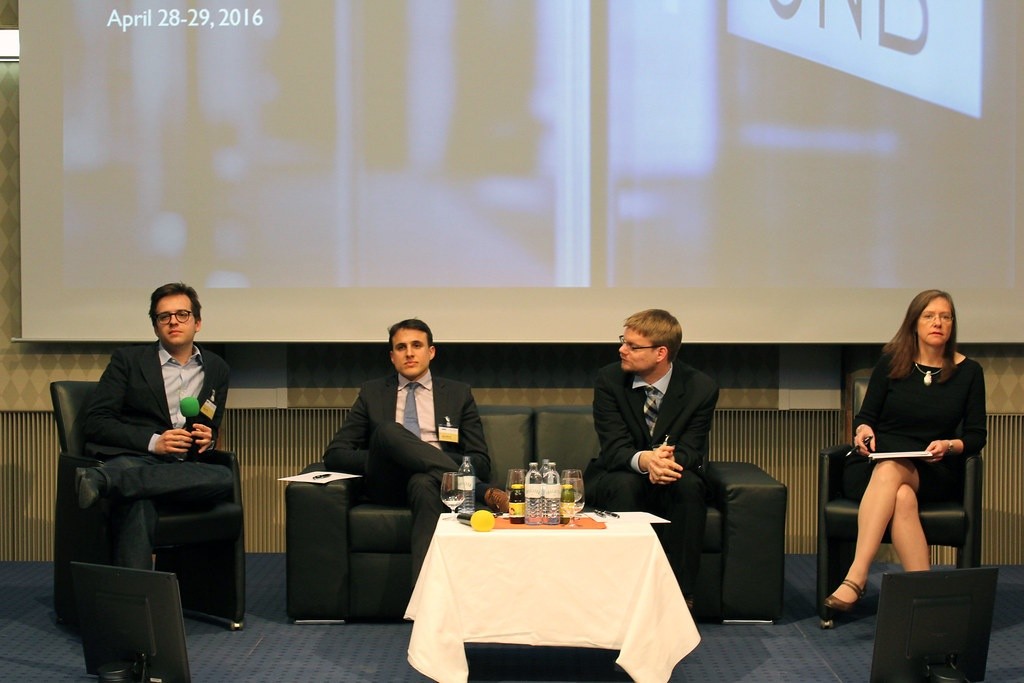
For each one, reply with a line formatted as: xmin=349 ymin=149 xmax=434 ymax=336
xmin=505 ymin=468 xmax=528 ymax=500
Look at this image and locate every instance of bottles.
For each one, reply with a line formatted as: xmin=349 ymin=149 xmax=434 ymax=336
xmin=457 ymin=456 xmax=475 ymax=514
xmin=509 ymin=459 xmax=573 ymax=525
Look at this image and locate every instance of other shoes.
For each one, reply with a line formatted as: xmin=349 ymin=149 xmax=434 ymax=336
xmin=485 ymin=487 xmax=510 ymax=515
xmin=825 ymin=578 xmax=869 ymax=611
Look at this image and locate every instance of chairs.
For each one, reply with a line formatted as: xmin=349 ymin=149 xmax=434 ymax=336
xmin=48 ymin=380 xmax=248 ymax=634
xmin=815 ymin=367 xmax=984 ymax=631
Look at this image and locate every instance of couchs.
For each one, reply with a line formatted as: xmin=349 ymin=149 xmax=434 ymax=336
xmin=283 ymin=404 xmax=788 ymax=625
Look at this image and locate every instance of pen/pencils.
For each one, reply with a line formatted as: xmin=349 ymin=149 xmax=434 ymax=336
xmin=313 ymin=474 xmax=332 ymax=479
xmin=603 ymin=511 xmax=620 ymax=518
xmin=846 ymin=436 xmax=873 ymax=457
xmin=593 ymin=509 xmax=606 ymax=518
xmin=663 ymin=436 xmax=670 ymax=445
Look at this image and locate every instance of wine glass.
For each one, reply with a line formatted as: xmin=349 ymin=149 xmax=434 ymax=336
xmin=440 ymin=472 xmax=465 ymax=520
xmin=560 ymin=469 xmax=585 ymax=528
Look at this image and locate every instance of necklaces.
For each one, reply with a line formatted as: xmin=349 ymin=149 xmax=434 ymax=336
xmin=914 ymin=359 xmax=942 ymax=386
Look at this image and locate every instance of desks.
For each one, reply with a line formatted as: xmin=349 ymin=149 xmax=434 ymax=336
xmin=431 ymin=512 xmax=659 ymax=683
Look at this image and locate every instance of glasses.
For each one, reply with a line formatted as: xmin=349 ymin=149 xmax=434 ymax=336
xmin=619 ymin=335 xmax=661 ymax=352
xmin=154 ymin=309 xmax=194 ymax=325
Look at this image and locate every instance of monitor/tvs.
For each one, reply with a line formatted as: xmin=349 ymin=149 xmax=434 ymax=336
xmin=69 ymin=561 xmax=192 ymax=683
xmin=869 ymin=566 xmax=1000 ymax=683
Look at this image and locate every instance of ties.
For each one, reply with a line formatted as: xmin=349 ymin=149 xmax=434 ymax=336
xmin=404 ymin=382 xmax=422 ymax=441
xmin=643 ymin=386 xmax=659 ymax=438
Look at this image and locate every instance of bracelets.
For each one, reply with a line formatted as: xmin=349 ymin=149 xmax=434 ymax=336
xmin=947 ymin=440 xmax=953 ymax=452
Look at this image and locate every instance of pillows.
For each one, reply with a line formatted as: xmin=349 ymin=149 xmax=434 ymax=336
xmin=536 ymin=411 xmax=602 ymax=478
xmin=480 ymin=414 xmax=532 ymax=492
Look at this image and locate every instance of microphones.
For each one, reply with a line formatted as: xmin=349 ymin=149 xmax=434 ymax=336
xmin=457 ymin=510 xmax=495 ymax=532
xmin=181 ymin=397 xmax=200 ymax=462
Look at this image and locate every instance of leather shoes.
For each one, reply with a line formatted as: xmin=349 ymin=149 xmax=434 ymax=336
xmin=74 ymin=468 xmax=105 ymax=510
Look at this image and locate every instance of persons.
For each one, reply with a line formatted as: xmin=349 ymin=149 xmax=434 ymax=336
xmin=824 ymin=289 xmax=987 ymax=610
xmin=322 ymin=319 xmax=509 ymax=592
xmin=583 ymin=309 xmax=720 ymax=612
xmin=76 ymin=283 xmax=233 ymax=636
